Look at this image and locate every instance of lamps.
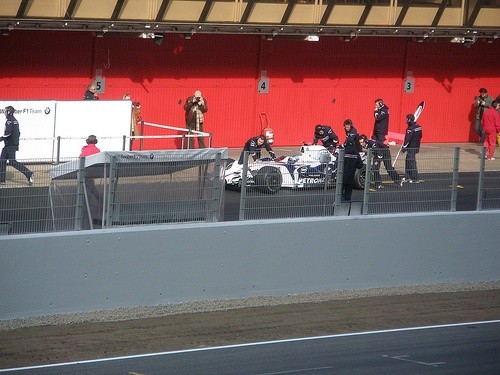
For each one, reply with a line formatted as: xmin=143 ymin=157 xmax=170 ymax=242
xmin=463 ymin=36 xmax=474 ymax=49
xmin=487 ymin=38 xmax=494 ymax=44
xmin=1 ymin=29 xmax=10 ymax=35
xmin=343 ymin=36 xmax=351 ymax=42
xmin=95 ymin=31 xmax=105 ymax=37
xmin=184 ymin=33 xmax=192 ymax=40
xmin=266 ymin=35 xmax=274 ymax=41
xmin=416 ymin=37 xmax=424 ymax=43
xmin=304 ymin=35 xmax=320 ymax=42
xmin=153 ymin=32 xmax=166 ymax=46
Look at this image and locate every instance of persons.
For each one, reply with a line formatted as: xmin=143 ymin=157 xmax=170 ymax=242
xmin=340 ymin=119 xmax=362 ymax=152
xmin=475 ymin=88 xmax=500 ymax=160
xmin=79 ymin=135 xmax=103 ymax=201
xmin=0 ymin=106 xmax=33 ymax=185
xmin=401 ymin=114 xmax=422 ymax=183
xmin=123 ymin=93 xmax=141 ymax=140
xmin=311 ymin=124 xmax=339 ymax=148
xmin=359 ymin=134 xmax=403 ymax=189
xmin=373 ymin=99 xmax=389 ymax=143
xmin=83 ymin=84 xmax=99 ymax=100
xmin=238 ymin=135 xmax=276 ymax=164
xmin=184 ymin=90 xmax=208 ymax=149
xmin=331 ymin=140 xmax=363 ymax=200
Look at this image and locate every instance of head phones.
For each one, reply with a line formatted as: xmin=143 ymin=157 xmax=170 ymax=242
xmin=315 ymin=126 xmax=326 ymax=135
xmin=7 ymin=110 xmax=11 ymax=115
xmin=86 ymin=139 xmax=97 ymax=144
xmin=407 ymin=119 xmax=411 ymax=123
xmin=379 ymin=102 xmax=383 ymax=107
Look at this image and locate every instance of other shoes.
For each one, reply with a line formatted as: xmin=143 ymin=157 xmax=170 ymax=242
xmin=485 ymin=156 xmax=495 ymax=160
xmin=0 ymin=181 xmax=5 ymax=185
xmin=397 ymin=178 xmax=405 ymax=190
xmin=28 ymin=173 xmax=33 ymax=186
xmin=409 ymin=180 xmax=420 ymax=184
xmin=374 ymin=185 xmax=384 ymax=190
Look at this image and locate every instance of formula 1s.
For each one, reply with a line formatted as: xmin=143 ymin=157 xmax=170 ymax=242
xmin=222 ymin=144 xmax=375 ymax=195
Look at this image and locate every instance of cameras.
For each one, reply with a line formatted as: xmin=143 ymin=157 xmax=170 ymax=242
xmin=195 ymin=97 xmax=200 ymax=100
